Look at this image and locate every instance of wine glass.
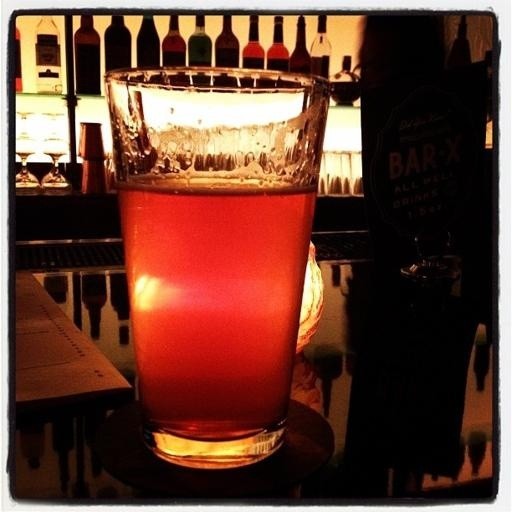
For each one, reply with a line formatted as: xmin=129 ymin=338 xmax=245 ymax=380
xmin=16 ymin=111 xmax=39 ymax=190
xmin=39 ymin=113 xmax=68 ymax=186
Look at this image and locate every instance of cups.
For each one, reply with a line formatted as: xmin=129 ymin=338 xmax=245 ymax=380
xmin=106 ymin=67 xmax=331 ymax=470
xmin=317 ymin=151 xmax=365 ymax=197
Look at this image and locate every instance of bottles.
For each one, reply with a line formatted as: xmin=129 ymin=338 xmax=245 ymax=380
xmin=52 ymin=416 xmax=74 ymax=495
xmin=137 ymin=15 xmax=160 ymax=67
xmin=14 ymin=27 xmax=22 ymax=94
xmin=468 ymin=432 xmax=487 ymax=476
xmin=310 ymin=15 xmax=331 ymax=79
xmin=20 ymin=421 xmax=45 ymax=468
xmin=266 ymin=16 xmax=290 ymax=72
xmin=85 ymin=413 xmax=106 ymax=479
xmin=473 ymin=335 xmax=490 ymax=392
xmin=35 ymin=16 xmax=63 ymax=94
xmin=215 ymin=16 xmax=239 ymax=67
xmin=81 ymin=273 xmax=107 ymax=340
xmin=290 ymin=15 xmax=312 ymax=72
xmin=445 ymin=14 xmax=473 ymax=68
xmin=188 ymin=15 xmax=212 ymax=67
xmin=110 ymin=273 xmax=130 ymax=345
xmin=104 ymin=15 xmax=131 ymax=74
xmin=162 ymin=16 xmax=186 ymax=67
xmin=329 ymin=55 xmax=361 ymax=106
xmin=40 ymin=272 xmax=68 ymax=315
xmin=314 ymin=346 xmax=343 ymax=418
xmin=74 ymin=15 xmax=101 ymax=95
xmin=242 ymin=15 xmax=265 ymax=69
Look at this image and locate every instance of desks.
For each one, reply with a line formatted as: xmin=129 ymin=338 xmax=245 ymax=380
xmin=16 ymin=270 xmax=135 ymax=412
